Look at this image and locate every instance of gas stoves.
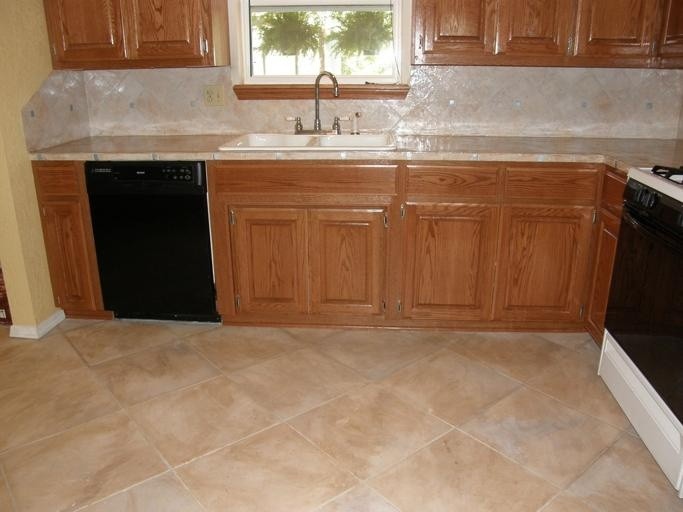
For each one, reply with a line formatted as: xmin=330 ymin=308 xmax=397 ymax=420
xmin=626 ymin=164 xmax=683 ymax=204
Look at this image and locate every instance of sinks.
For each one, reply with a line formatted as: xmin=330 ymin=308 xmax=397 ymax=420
xmin=217 ymin=133 xmax=312 ymax=151
xmin=310 ymin=130 xmax=398 ymax=151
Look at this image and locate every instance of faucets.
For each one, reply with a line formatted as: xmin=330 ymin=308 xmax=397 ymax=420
xmin=314 ymin=69 xmax=339 ymax=132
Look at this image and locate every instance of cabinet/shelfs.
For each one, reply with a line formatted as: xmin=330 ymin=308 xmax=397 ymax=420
xmin=208 ymin=161 xmax=398 ymax=330
xmin=583 ymin=166 xmax=630 ymax=350
xmin=31 ymin=160 xmax=105 ymax=321
xmin=410 ymin=1 xmax=683 ymax=70
xmin=43 ymin=0 xmax=231 ymax=70
xmin=398 ymin=163 xmax=608 ymax=334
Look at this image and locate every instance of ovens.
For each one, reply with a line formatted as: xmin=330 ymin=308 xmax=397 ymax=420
xmin=83 ymin=159 xmax=221 ymax=323
xmin=602 ymin=176 xmax=683 ymax=426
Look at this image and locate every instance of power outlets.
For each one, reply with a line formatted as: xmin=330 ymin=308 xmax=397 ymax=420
xmin=202 ymin=84 xmax=226 ymax=108
